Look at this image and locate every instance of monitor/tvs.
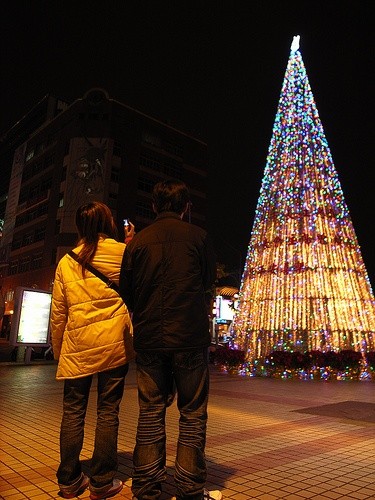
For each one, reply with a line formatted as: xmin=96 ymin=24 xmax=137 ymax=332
xmin=10 ymin=286 xmax=52 ymax=347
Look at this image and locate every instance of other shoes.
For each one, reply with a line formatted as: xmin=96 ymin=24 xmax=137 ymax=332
xmin=62 ymin=475 xmax=89 ymax=498
xmin=203 ymin=489 xmax=222 ymax=500
xmin=89 ymin=479 xmax=123 ymax=500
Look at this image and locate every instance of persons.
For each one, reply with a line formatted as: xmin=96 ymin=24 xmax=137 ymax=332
xmin=51 ymin=201 xmax=137 ymax=500
xmin=120 ymin=181 xmax=218 ymax=500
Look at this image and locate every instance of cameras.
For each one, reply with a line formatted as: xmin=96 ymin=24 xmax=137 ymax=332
xmin=123 ymin=219 xmax=131 ymax=233
